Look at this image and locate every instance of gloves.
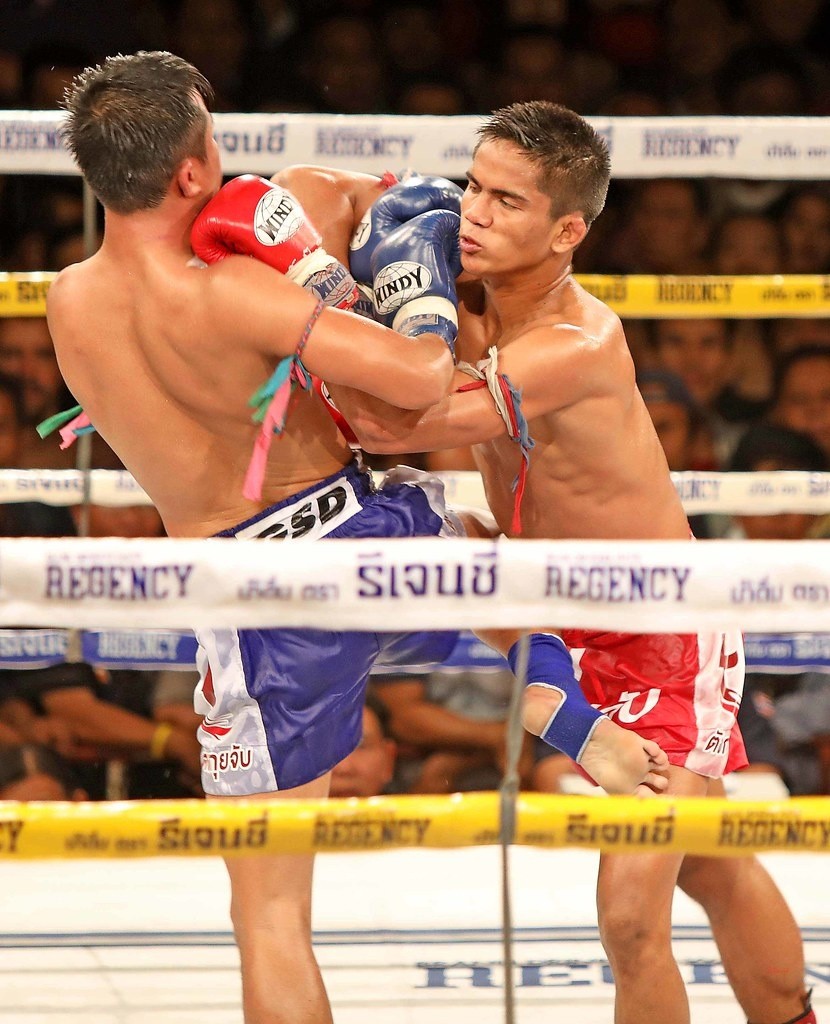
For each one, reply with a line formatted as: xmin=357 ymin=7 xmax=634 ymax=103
xmin=189 ymin=174 xmax=323 ymax=277
xmin=304 ymin=373 xmax=363 ymax=451
xmin=369 ymin=207 xmax=465 ymax=342
xmin=349 ymin=173 xmax=466 ymax=284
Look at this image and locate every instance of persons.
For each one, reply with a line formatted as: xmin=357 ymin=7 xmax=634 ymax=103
xmin=46 ymin=50 xmax=671 ymax=1024
xmin=187 ymin=99 xmax=816 ymax=1024
xmin=0 ymin=0 xmax=830 ymax=803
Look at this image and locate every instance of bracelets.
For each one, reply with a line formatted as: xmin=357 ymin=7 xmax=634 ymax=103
xmin=149 ymin=722 xmax=173 ymax=759
